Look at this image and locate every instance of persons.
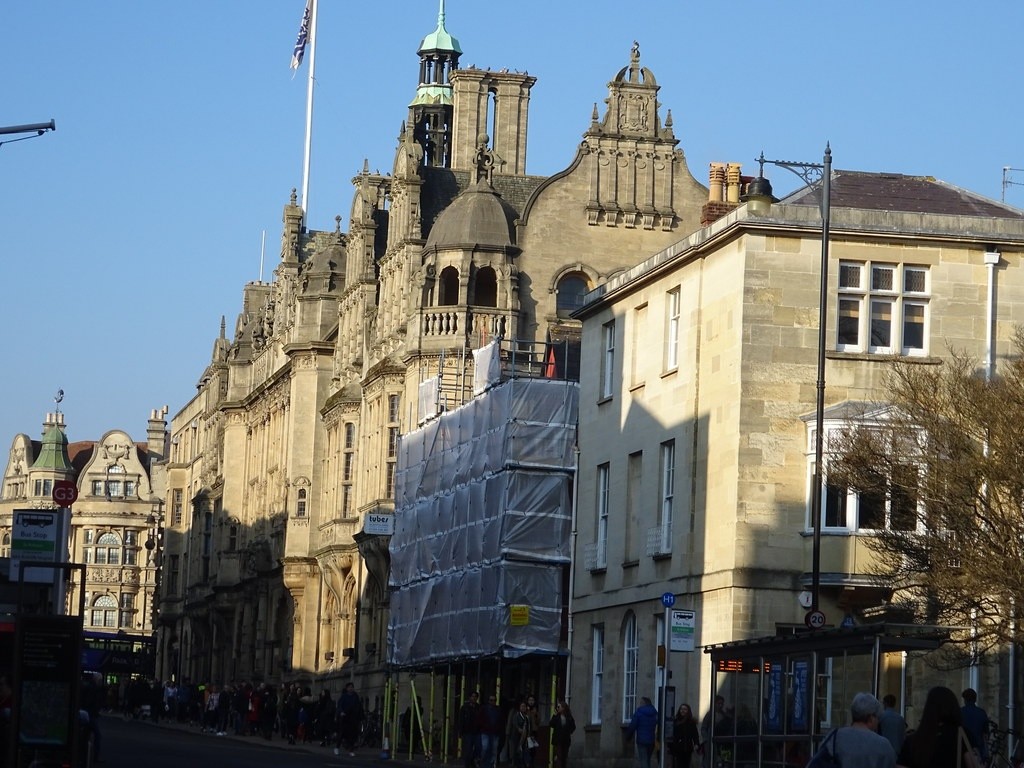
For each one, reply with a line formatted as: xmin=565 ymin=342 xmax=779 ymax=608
xmin=402 ymin=696 xmax=424 ymax=751
xmin=624 ymin=697 xmax=657 ymax=768
xmin=818 ymin=693 xmax=897 ymax=768
xmin=667 ymin=704 xmax=701 ymax=768
xmin=700 ymin=696 xmax=797 ymax=768
xmin=897 ymin=686 xmax=979 ymax=768
xmin=0 ymin=671 xmax=106 ymax=768
xmin=959 ymin=688 xmax=990 ymax=763
xmin=103 ymin=679 xmax=365 ymax=756
xmin=458 ymin=692 xmax=576 ymax=768
xmin=878 ymin=694 xmax=906 ymax=755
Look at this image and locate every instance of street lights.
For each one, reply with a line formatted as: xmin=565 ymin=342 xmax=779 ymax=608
xmin=737 ymin=152 xmax=831 ymax=768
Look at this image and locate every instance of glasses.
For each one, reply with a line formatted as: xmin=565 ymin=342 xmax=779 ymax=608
xmin=557 ymin=706 xmax=560 ymax=708
xmin=471 ymin=695 xmax=478 ymax=697
xmin=488 ymin=699 xmax=496 ymax=702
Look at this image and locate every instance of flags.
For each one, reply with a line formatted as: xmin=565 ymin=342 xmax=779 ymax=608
xmin=289 ymin=0 xmax=313 ymax=80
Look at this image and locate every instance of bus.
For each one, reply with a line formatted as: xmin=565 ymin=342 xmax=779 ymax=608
xmin=81 ymin=631 xmax=156 ymax=714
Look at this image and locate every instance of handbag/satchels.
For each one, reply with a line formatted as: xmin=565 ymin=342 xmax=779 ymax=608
xmin=805 ymin=728 xmax=839 ymax=768
xmin=527 ymin=736 xmax=539 ymax=748
xmin=248 ymin=699 xmax=252 ymax=711
xmin=957 ymin=726 xmax=982 ymax=768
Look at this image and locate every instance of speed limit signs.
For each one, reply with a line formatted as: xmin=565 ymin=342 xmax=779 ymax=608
xmin=804 ymin=610 xmax=826 ymax=628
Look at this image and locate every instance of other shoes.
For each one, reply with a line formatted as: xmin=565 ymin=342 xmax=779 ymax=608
xmin=201 ymin=724 xmax=330 ymax=747
xmin=334 ymin=748 xmax=340 ymax=755
xmin=349 ymin=751 xmax=356 ymax=757
